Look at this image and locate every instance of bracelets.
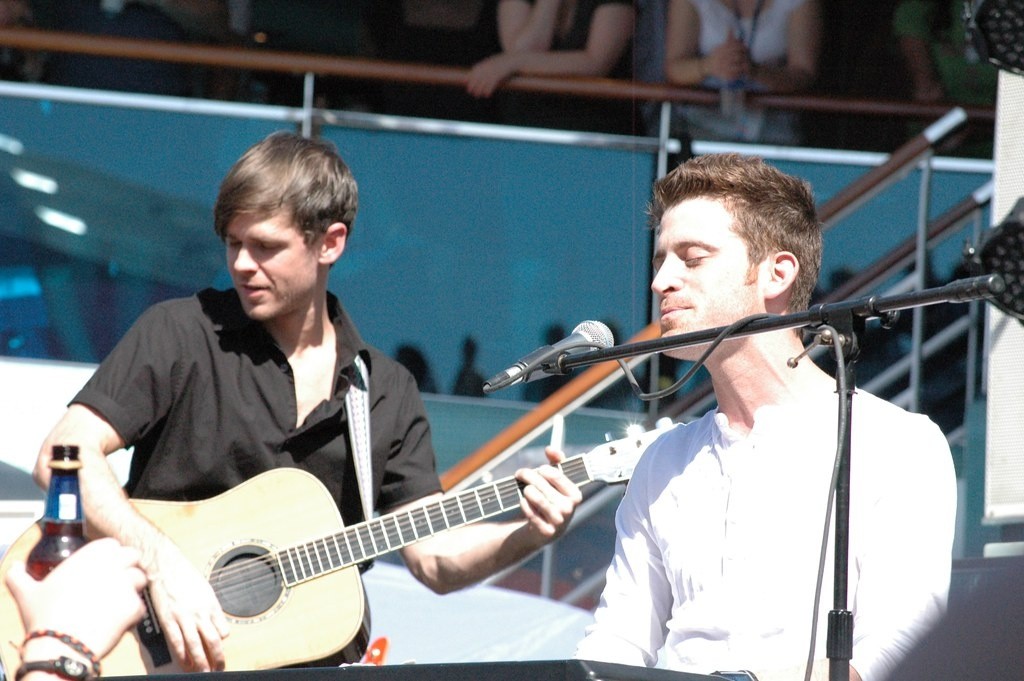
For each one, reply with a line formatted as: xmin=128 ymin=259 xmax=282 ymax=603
xmin=23 ymin=629 xmax=101 ymax=675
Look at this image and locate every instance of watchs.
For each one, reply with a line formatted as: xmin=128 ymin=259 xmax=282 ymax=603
xmin=15 ymin=657 xmax=93 ymax=681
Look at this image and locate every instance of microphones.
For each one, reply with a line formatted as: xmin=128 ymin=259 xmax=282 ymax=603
xmin=483 ymin=320 xmax=615 ymax=395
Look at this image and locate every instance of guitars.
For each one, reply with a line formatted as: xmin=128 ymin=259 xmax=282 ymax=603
xmin=0 ymin=409 xmax=695 ymax=681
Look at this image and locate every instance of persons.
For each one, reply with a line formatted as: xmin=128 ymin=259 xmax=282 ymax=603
xmin=33 ymin=130 xmax=583 ymax=675
xmin=6 ymin=539 xmax=148 ymax=681
xmin=573 ymin=155 xmax=957 ymax=681
xmin=1 ymin=1 xmax=1023 ymax=144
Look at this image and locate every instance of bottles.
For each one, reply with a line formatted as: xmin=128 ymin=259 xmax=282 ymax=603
xmin=25 ymin=444 xmax=89 ymax=581
xmin=720 ymin=31 xmax=746 ymax=113
